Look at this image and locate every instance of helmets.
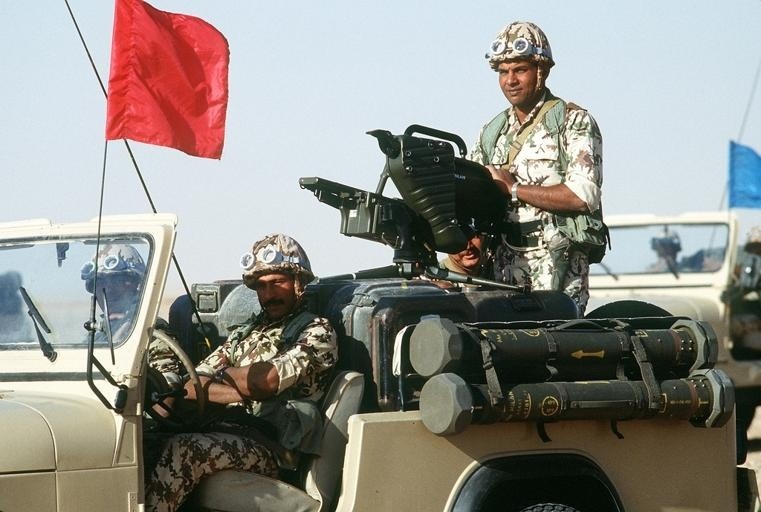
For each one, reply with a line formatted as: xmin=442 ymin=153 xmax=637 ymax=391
xmin=489 ymin=21 xmax=556 ymax=72
xmin=240 ymin=233 xmax=315 ymax=290
xmin=80 ymin=241 xmax=146 ymax=292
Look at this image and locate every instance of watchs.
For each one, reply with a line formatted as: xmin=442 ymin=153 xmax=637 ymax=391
xmin=215 ymin=367 xmax=228 ymax=383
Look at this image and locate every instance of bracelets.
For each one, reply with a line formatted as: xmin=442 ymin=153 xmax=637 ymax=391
xmin=511 ymin=181 xmax=522 ymax=207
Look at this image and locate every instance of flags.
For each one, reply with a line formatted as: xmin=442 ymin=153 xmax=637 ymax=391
xmin=106 ymin=0 xmax=230 ymax=160
xmin=728 ymin=140 xmax=761 ymax=210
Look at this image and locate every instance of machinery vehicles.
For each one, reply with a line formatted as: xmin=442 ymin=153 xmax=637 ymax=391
xmin=0 ymin=210 xmax=760 ymax=512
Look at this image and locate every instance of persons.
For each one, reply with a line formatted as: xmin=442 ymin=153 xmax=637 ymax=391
xmin=730 ymin=225 xmax=761 ymax=338
xmin=80 ymin=242 xmax=179 ymax=432
xmin=143 ymin=234 xmax=337 ymax=511
xmin=466 ymin=20 xmax=611 ymax=318
xmin=644 ymin=231 xmax=698 ymax=274
xmin=412 ymin=236 xmax=492 ymax=293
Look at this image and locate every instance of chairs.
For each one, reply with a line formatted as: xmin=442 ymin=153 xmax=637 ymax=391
xmin=194 ymin=370 xmax=365 ymax=512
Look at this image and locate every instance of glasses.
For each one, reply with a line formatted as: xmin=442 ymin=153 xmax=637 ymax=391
xmin=81 ymin=255 xmax=124 ymax=277
xmin=239 ymin=247 xmax=283 ymax=269
xmin=490 ymin=37 xmax=534 ymax=56
